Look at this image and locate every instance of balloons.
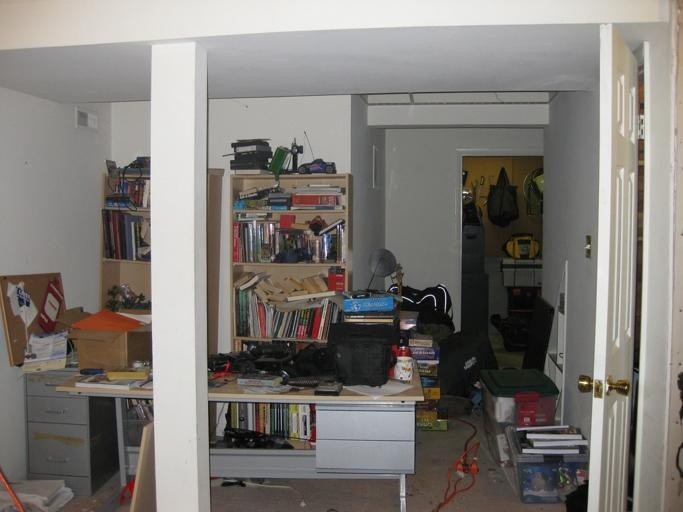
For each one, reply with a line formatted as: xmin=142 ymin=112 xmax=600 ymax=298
xmin=233 ymin=213 xmax=345 ymax=263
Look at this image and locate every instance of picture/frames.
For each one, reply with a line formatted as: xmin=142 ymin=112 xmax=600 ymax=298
xmin=372 ymin=145 xmax=382 ymax=191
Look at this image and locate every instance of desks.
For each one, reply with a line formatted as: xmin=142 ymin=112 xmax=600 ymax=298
xmin=54 ymin=346 xmax=425 ymax=511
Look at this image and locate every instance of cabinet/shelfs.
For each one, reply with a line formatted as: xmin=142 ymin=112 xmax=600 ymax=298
xmin=101 ymin=176 xmax=352 ymax=357
xmin=25 ymin=368 xmax=119 ymax=497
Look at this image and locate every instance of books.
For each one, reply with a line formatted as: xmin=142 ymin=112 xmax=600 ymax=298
xmin=234 ymin=269 xmax=345 ymax=351
xmin=77 ymin=370 xmax=142 ymax=391
xmin=0 ymin=477 xmax=74 ymax=512
xmin=400 ymin=310 xmax=439 ymax=386
xmin=237 ymin=373 xmax=283 ymax=387
xmin=343 ymin=292 xmax=398 ymax=325
xmin=230 ymin=402 xmax=312 ymax=439
xmin=102 ymin=209 xmax=150 ymax=262
xmin=234 ymin=184 xmax=345 ymax=209
xmin=106 ymin=179 xmax=149 ymax=208
xmin=509 ymin=416 xmax=589 ymax=459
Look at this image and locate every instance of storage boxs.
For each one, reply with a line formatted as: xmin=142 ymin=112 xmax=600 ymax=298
xmin=477 ymin=366 xmax=590 ymax=503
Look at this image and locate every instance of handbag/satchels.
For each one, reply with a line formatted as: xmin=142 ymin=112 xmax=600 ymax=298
xmin=488 ymin=167 xmax=519 ymax=226
xmin=328 ymin=323 xmax=393 ymax=387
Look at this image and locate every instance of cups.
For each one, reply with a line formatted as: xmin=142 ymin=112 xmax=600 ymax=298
xmin=394 ymin=356 xmax=414 ymax=381
